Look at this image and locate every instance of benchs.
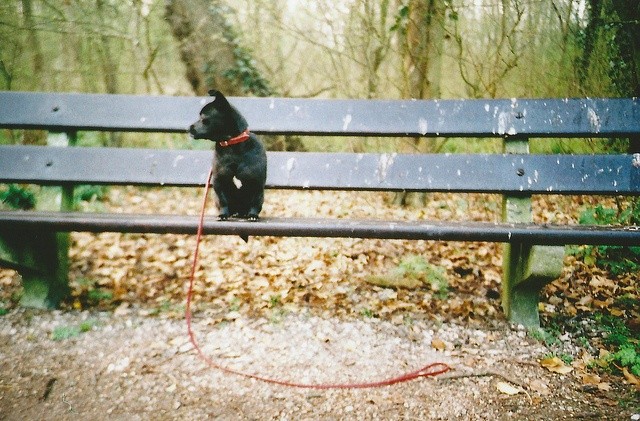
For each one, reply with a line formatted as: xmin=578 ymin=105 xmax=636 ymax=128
xmin=1 ymin=90 xmax=637 ymax=330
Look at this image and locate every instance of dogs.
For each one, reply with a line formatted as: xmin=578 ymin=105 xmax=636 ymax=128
xmin=188 ymin=89 xmax=268 ymax=222
